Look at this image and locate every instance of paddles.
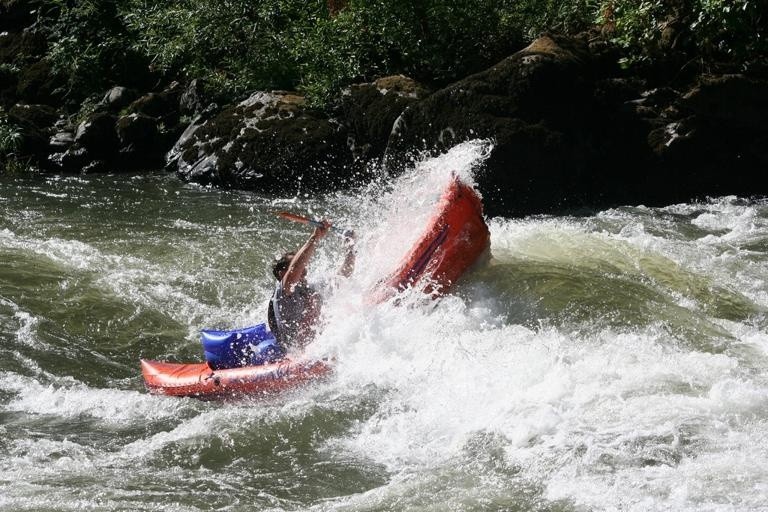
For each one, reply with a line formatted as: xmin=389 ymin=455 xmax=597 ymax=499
xmin=278 ymin=212 xmax=355 ymax=237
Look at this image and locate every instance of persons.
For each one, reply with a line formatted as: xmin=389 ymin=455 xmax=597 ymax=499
xmin=272 ymin=227 xmax=358 ymax=354
